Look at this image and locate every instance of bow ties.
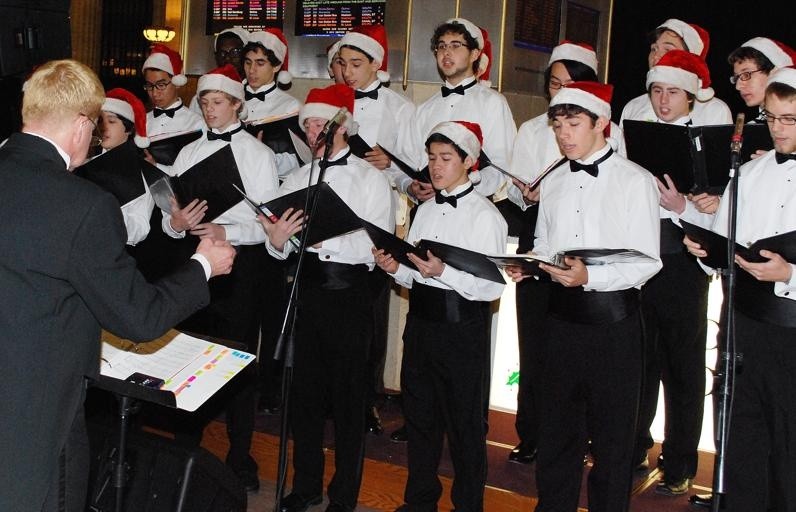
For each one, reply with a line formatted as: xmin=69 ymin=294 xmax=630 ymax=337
xmin=440 ymin=85 xmax=465 ymax=97
xmin=245 ymin=89 xmax=265 ymax=101
xmin=207 ymin=131 xmax=231 ymax=143
xmin=354 ymin=89 xmax=378 ymax=101
xmin=318 ymin=157 xmax=347 ymax=168
xmin=775 ymin=151 xmax=796 ymax=164
xmin=153 ymin=108 xmax=175 ymax=118
xmin=570 ymin=160 xmax=599 ymax=178
xmin=435 ymin=192 xmax=457 ymax=209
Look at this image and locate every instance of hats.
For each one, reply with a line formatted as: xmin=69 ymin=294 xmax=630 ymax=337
xmin=247 ymin=28 xmax=291 ymax=84
xmin=656 ymin=19 xmax=709 ymax=60
xmin=427 ymin=121 xmax=483 ymax=184
xmin=548 ymin=82 xmax=613 ymax=138
xmin=196 ymin=64 xmax=245 ymax=103
xmin=547 ymin=40 xmax=598 ymax=76
xmin=214 ymin=27 xmax=250 ymax=51
xmin=646 ymin=50 xmax=714 ymax=102
xmin=99 ymin=88 xmax=150 ymax=148
xmin=142 ymin=45 xmax=187 ymax=86
xmin=299 ymin=83 xmax=359 ymax=136
xmin=444 ymin=17 xmax=492 ymax=88
xmin=339 ymin=24 xmax=390 ymax=82
xmin=766 ymin=66 xmax=796 ymax=89
xmin=741 ymin=37 xmax=796 ymax=69
xmin=326 ymin=40 xmax=340 ymax=71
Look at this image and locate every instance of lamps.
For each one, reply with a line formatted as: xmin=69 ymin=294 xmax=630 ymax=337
xmin=142 ymin=26 xmax=174 ymax=47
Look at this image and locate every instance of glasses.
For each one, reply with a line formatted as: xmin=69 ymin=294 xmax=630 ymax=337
xmin=143 ymin=83 xmax=170 ymax=90
xmin=434 ymin=42 xmax=467 ymax=51
xmin=761 ymin=109 xmax=796 ymax=125
xmin=216 ymin=48 xmax=241 ymax=58
xmin=549 ymin=81 xmax=567 ymax=90
xmin=730 ymin=70 xmax=760 ymax=84
xmin=80 ymin=113 xmax=103 ymax=146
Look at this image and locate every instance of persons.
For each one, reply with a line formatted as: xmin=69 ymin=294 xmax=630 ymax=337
xmin=624 ymin=51 xmax=719 ymax=497
xmin=688 ymin=35 xmax=795 ymax=214
xmin=506 ymin=83 xmax=663 ymax=512
xmin=326 ymin=43 xmax=347 ymax=87
xmin=326 ymin=25 xmax=422 ymax=440
xmin=163 ymin=62 xmax=279 ymax=490
xmin=391 ymin=18 xmax=519 ymax=441
xmin=396 ymin=119 xmax=509 ymax=512
xmin=618 ymin=19 xmax=732 ymax=127
xmin=721 ymin=68 xmax=795 ymax=512
xmin=0 ymin=60 xmax=236 ymax=511
xmin=238 ymin=29 xmax=301 ymax=413
xmin=141 ymin=46 xmax=205 ymax=175
xmin=511 ymin=40 xmax=627 ymax=465
xmin=96 ymin=88 xmax=155 ymax=248
xmin=214 ymin=26 xmax=247 ymax=81
xmin=277 ymin=83 xmax=396 ymax=512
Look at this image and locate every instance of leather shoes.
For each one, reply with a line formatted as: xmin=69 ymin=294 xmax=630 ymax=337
xmin=636 ymin=454 xmax=649 ymax=470
xmin=689 ymin=493 xmax=713 ymax=506
xmin=657 ymin=454 xmax=664 ymax=469
xmin=390 ymin=426 xmax=405 ymax=442
xmin=508 ymin=441 xmax=537 ymax=464
xmin=276 ymin=491 xmax=322 ymax=512
xmin=654 ymin=478 xmax=689 ymax=495
xmin=257 ymin=398 xmax=282 ymax=414
xmin=246 ymin=477 xmax=260 ymax=494
xmin=367 ymin=407 xmax=384 ymax=434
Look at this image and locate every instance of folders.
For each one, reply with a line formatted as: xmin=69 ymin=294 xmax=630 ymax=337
xmin=70 ymin=141 xmax=162 ymax=204
xmin=474 ymin=150 xmax=491 ymax=170
xmin=488 ymin=157 xmax=565 ymax=191
xmin=360 ymin=217 xmax=507 ymax=289
xmin=144 ymin=161 xmax=247 ymax=226
xmin=623 ymin=119 xmax=774 ymax=197
xmin=144 ymin=129 xmax=203 ymax=166
xmin=234 ymin=184 xmax=365 ymax=253
xmin=378 ymin=142 xmax=431 ymax=188
xmin=677 ymin=217 xmax=796 ymax=277
xmin=344 ymin=132 xmax=372 ymax=161
xmin=240 ymin=114 xmax=311 ymax=169
xmin=488 ymin=247 xmax=647 ymax=277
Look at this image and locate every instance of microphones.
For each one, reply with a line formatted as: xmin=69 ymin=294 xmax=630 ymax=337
xmin=728 ymin=113 xmax=748 ymax=154
xmin=310 ymin=107 xmax=351 ymax=150
